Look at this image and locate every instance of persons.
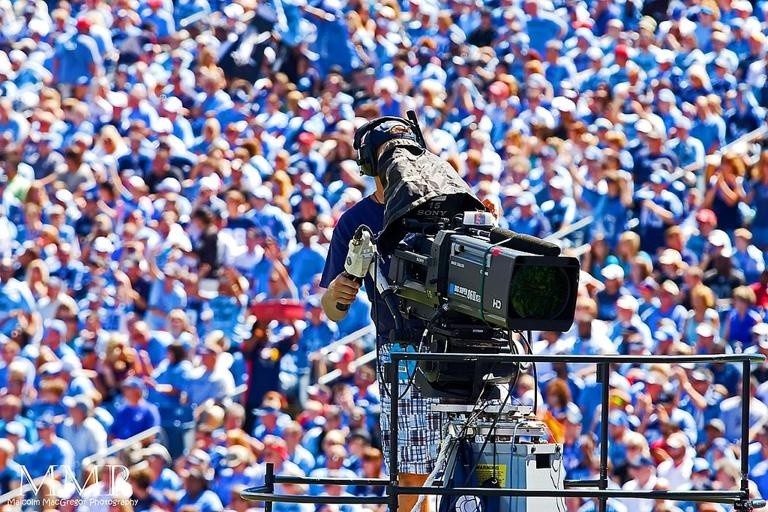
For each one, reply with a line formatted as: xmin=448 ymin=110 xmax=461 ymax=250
xmin=0 ymin=1 xmax=768 ymax=511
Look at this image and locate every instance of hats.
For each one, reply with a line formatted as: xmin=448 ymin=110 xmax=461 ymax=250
xmin=361 ymin=120 xmax=416 ymax=149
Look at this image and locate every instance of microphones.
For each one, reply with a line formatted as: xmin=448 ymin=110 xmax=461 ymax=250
xmin=406 ymin=110 xmax=427 ymax=149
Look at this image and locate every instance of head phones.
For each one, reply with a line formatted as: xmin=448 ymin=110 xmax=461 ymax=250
xmin=353 ymin=115 xmax=431 ymax=177
